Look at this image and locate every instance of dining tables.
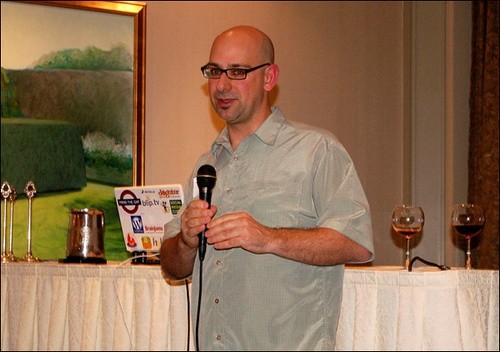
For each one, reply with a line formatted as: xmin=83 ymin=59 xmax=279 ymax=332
xmin=1 ymin=255 xmax=499 ymax=352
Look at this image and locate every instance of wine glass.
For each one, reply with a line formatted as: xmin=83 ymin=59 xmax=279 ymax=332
xmin=391 ymin=206 xmax=425 ymax=272
xmin=451 ymin=204 xmax=486 ymax=271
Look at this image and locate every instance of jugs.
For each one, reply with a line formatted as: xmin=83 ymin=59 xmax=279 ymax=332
xmin=62 ymin=207 xmax=108 ymax=264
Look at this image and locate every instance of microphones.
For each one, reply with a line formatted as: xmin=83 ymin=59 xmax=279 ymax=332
xmin=197 ymin=164 xmax=217 ymax=262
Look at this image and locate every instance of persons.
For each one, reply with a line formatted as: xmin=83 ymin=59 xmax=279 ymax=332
xmin=157 ymin=23 xmax=378 ymax=350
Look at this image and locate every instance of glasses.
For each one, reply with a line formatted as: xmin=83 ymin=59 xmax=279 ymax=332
xmin=201 ymin=62 xmax=271 ymax=80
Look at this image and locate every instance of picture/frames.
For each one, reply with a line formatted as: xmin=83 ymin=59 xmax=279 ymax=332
xmin=0 ymin=0 xmax=146 ymax=262
